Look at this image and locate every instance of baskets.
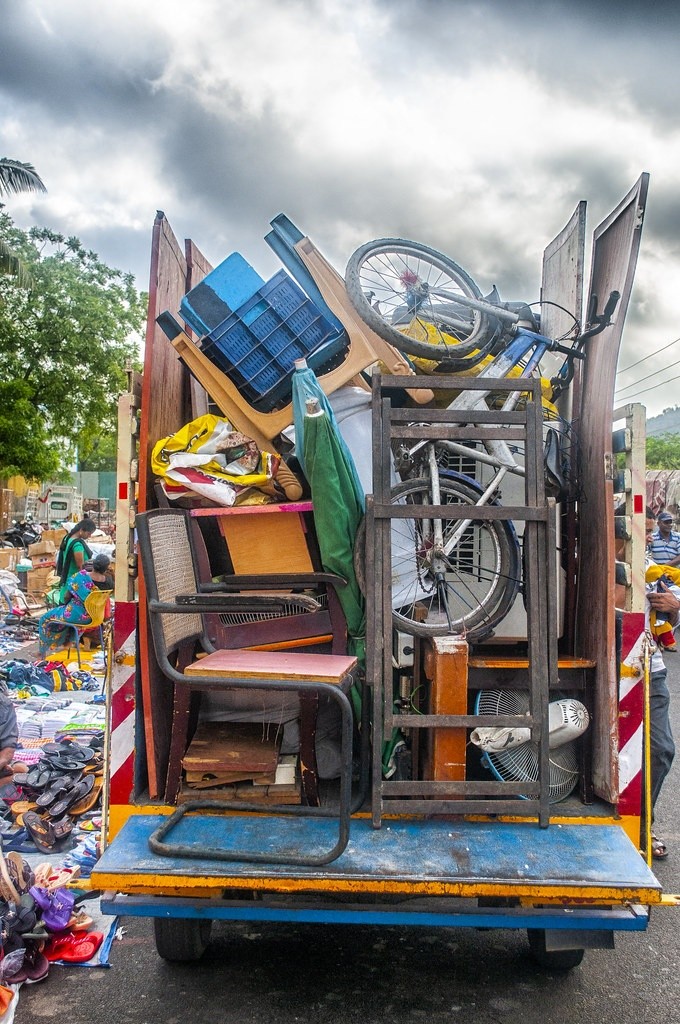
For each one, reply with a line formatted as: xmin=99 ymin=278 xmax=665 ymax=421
xmin=488 ymin=394 xmax=589 ymax=504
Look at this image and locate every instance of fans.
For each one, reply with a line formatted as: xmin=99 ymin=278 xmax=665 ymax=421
xmin=468 ymin=677 xmax=591 ymax=804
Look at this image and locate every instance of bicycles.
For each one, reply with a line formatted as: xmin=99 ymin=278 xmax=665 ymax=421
xmin=343 ymin=235 xmax=624 ymax=643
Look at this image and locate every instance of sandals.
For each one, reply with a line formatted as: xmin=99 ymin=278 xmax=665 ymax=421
xmin=640 ymin=830 xmax=669 ymax=859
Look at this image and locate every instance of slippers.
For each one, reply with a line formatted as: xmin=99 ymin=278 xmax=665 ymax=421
xmin=0 ymin=731 xmax=111 ymax=987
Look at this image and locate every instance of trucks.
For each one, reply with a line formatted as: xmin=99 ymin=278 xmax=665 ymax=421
xmin=38 ymin=485 xmax=83 ymax=530
xmin=75 ymin=169 xmax=663 ymax=977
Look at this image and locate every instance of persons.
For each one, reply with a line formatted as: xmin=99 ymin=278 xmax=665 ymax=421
xmin=613 ymin=500 xmax=680 ymax=859
xmin=29 ymin=553 xmax=118 ymax=667
xmin=647 ymin=512 xmax=680 ymax=652
xmin=0 ymin=692 xmax=30 ymax=788
xmin=59 ymin=518 xmax=97 ymax=611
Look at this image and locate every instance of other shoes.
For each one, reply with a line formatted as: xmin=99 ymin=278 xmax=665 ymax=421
xmin=664 ymin=645 xmax=677 ymax=652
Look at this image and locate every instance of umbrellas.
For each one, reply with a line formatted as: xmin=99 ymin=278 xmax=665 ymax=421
xmin=287 ymin=355 xmax=412 ymax=802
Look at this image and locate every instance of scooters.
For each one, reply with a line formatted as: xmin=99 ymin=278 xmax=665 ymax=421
xmin=0 ymin=512 xmax=44 ymax=549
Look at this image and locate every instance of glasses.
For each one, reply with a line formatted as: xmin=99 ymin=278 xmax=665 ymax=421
xmin=664 ymin=520 xmax=672 ymax=524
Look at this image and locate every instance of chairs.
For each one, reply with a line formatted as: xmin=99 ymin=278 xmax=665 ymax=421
xmin=41 ymin=588 xmax=115 ymax=670
xmin=132 ymin=507 xmax=374 ymax=866
xmin=154 ymin=213 xmax=437 ymax=502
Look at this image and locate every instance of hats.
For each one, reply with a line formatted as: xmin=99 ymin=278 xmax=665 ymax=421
xmin=658 ymin=513 xmax=673 ymax=521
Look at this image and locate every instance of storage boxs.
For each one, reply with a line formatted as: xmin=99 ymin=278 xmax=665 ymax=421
xmin=0 ymin=528 xmax=69 ymax=597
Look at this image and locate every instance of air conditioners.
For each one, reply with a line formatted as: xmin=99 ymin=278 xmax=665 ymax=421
xmin=420 ymin=416 xmax=566 ymax=646
